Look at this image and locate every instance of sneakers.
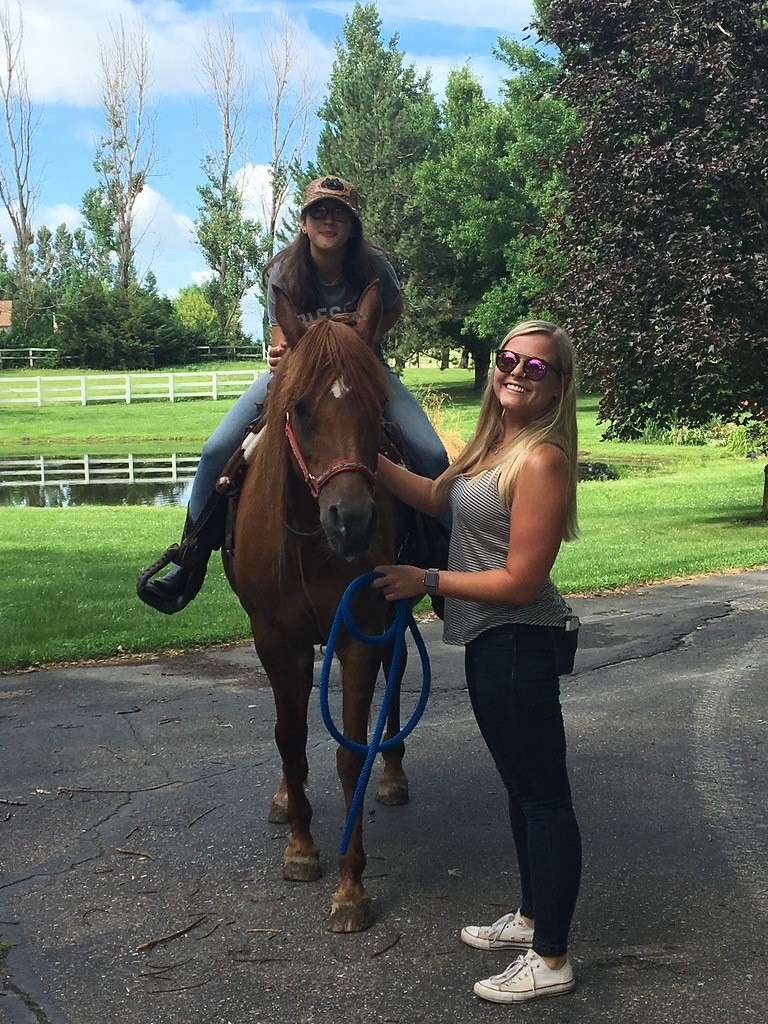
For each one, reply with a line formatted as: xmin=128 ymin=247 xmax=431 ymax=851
xmin=474 ymin=950 xmax=576 ymax=1004
xmin=460 ymin=908 xmax=535 ymax=952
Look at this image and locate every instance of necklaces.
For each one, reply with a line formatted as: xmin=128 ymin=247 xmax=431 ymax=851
xmin=494 ymin=445 xmax=504 ymax=454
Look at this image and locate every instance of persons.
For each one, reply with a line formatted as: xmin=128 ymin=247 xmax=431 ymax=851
xmin=370 ymin=320 xmax=582 ymax=1005
xmin=154 ymin=175 xmax=450 ymax=624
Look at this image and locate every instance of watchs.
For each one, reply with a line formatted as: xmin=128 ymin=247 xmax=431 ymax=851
xmin=423 ymin=567 xmax=439 ymax=596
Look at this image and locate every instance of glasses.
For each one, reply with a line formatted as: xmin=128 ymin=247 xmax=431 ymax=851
xmin=310 ymin=197 xmax=353 ymax=223
xmin=494 ymin=348 xmax=560 ymax=381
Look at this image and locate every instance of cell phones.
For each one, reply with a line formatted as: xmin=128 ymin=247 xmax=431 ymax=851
xmin=565 ymin=616 xmax=578 ymax=631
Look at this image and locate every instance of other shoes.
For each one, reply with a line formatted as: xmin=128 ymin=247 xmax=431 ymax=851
xmin=426 ymin=548 xmax=453 ymax=621
xmin=145 ymin=566 xmax=206 ymax=603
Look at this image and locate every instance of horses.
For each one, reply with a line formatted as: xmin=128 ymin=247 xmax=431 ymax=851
xmin=222 ymin=275 xmax=438 ymax=936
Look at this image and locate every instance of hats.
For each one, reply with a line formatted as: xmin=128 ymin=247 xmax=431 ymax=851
xmin=299 ymin=175 xmax=359 ymax=215
